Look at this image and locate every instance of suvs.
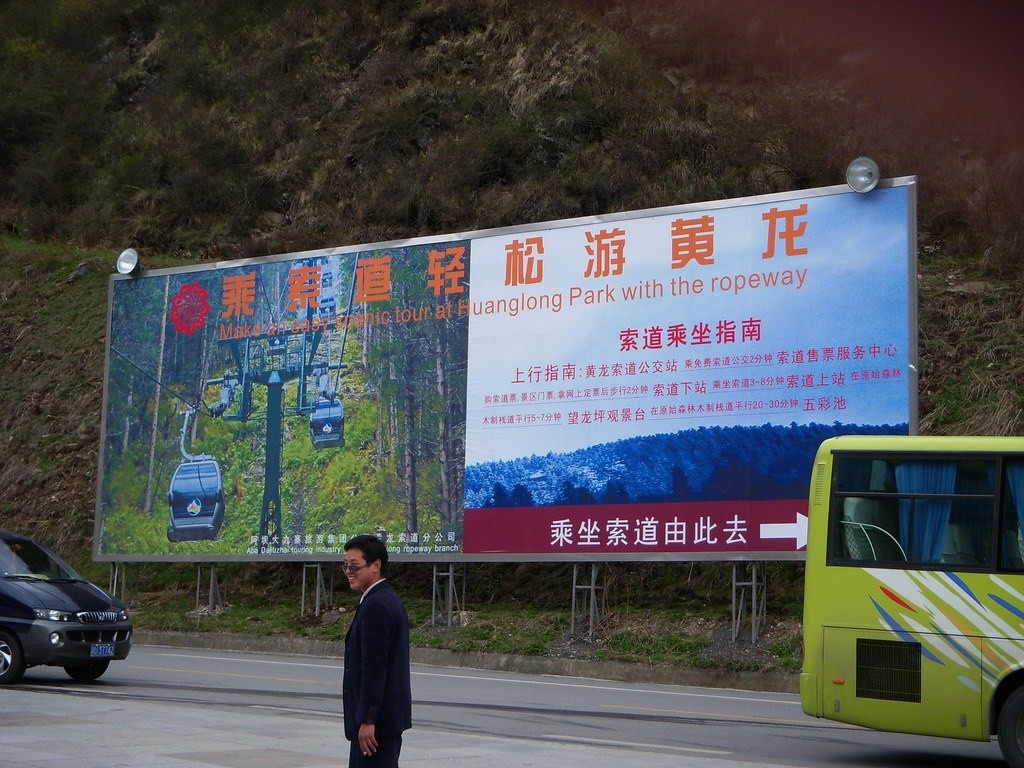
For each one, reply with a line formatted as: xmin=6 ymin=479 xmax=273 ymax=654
xmin=0 ymin=530 xmax=133 ymax=685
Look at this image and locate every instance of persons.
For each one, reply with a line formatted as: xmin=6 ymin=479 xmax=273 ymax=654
xmin=342 ymin=535 xmax=411 ymax=768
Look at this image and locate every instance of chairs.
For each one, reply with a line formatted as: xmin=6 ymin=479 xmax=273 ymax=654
xmin=843 ymin=496 xmax=900 ymax=559
xmin=1004 ymin=529 xmax=1024 ymax=569
xmin=939 ymin=524 xmax=978 ymax=562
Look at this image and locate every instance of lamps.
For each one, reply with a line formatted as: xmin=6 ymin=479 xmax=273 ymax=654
xmin=117 ymin=248 xmax=141 ymax=275
xmin=848 ymin=157 xmax=881 ymax=193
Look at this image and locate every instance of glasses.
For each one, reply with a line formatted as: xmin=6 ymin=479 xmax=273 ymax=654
xmin=342 ymin=561 xmax=373 ymax=571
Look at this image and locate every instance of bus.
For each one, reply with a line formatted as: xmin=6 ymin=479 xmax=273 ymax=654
xmin=798 ymin=434 xmax=1024 ymax=768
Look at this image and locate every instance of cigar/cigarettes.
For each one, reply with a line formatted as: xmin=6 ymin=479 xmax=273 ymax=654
xmin=363 ymin=751 xmax=366 ymax=756
